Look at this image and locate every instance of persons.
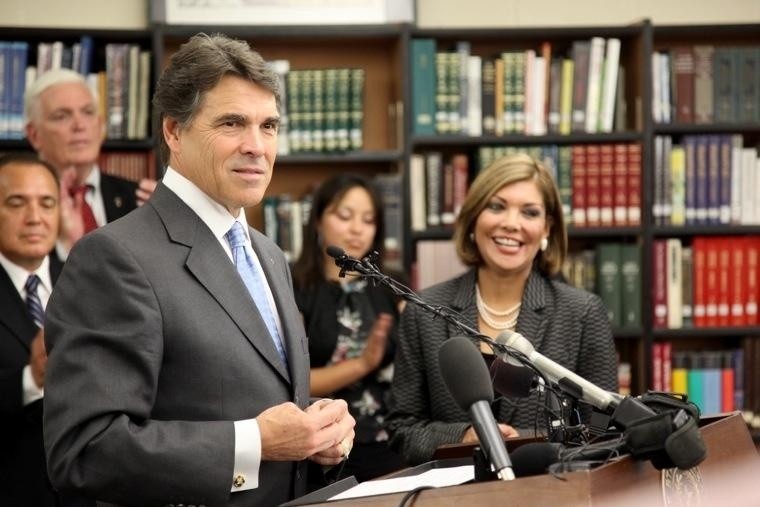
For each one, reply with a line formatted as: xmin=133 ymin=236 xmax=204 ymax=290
xmin=293 ymin=175 xmax=410 ymax=481
xmin=40 ymin=32 xmax=356 ymax=505
xmin=0 ymin=154 xmax=68 ymax=505
xmin=382 ymin=154 xmax=619 ymax=468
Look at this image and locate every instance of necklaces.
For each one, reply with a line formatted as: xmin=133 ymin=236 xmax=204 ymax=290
xmin=475 ymin=284 xmax=522 ymax=330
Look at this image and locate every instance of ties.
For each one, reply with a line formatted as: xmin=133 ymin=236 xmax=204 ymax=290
xmin=25 ymin=274 xmax=46 ymax=331
xmin=226 ymin=222 xmax=291 ymax=375
xmin=67 ymin=184 xmax=98 ymax=235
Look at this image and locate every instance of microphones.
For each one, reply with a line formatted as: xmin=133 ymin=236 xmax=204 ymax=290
xmin=489 ymin=355 xmax=535 ymax=400
xmin=440 ymin=337 xmax=515 ymax=481
xmin=510 ymin=439 xmax=626 ymax=479
xmin=326 ymin=244 xmax=560 ymax=414
xmin=492 ymin=331 xmax=621 ymax=414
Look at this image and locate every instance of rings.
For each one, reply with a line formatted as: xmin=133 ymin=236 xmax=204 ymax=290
xmin=342 ymin=450 xmax=351 ymax=457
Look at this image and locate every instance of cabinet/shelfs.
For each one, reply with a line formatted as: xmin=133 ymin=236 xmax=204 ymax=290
xmin=0 ymin=23 xmax=411 ymax=280
xmin=411 ymin=19 xmax=759 ymax=442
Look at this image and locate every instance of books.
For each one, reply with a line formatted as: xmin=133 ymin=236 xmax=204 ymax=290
xmin=655 ymin=133 xmax=758 ymax=227
xmin=417 ymin=243 xmax=643 ymax=334
xmin=652 ymin=235 xmax=760 ymax=330
xmin=97 ymin=152 xmax=148 ymax=183
xmin=616 ymin=363 xmax=631 ymax=402
xmin=435 ymin=37 xmax=626 ymax=138
xmin=2 ymin=38 xmax=151 ymax=142
xmin=652 ymin=341 xmax=760 ymax=412
xmin=651 ymin=43 xmax=759 ymax=124
xmin=266 ymin=60 xmax=364 ymax=156
xmin=263 ymin=175 xmax=407 ymax=278
xmin=408 ymin=144 xmax=641 ymax=233
